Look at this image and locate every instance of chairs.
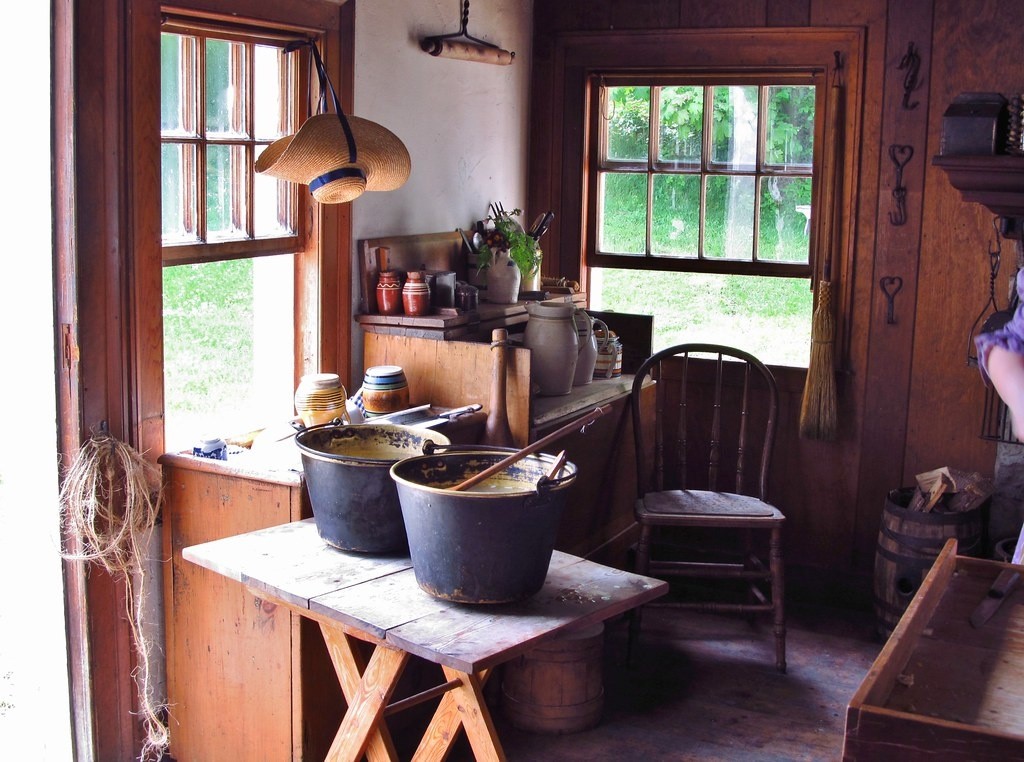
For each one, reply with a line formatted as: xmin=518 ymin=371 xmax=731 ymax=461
xmin=627 ymin=343 xmax=788 ymax=669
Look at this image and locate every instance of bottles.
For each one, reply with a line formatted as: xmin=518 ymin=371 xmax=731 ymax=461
xmin=594 ymin=329 xmax=622 ymax=379
xmin=191 ymin=437 xmax=228 ymax=462
xmin=992 ymin=536 xmax=1019 ymax=567
xmin=363 ymin=366 xmax=409 ymax=418
xmin=487 ymin=249 xmax=521 ymax=303
xmin=294 ymin=373 xmax=347 ymax=428
xmin=522 ymin=301 xmax=591 ymax=397
xmin=401 ymin=271 xmax=431 ymax=317
xmin=376 ymin=270 xmax=401 ymax=315
xmin=457 ymin=286 xmax=479 ymax=312
xmin=574 ymin=309 xmax=610 ymax=385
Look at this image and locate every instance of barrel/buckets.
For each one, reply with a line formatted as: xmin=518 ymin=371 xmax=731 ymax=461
xmin=390 ymin=451 xmax=578 ymax=602
xmin=874 ymin=488 xmax=992 ymax=644
xmin=500 ymin=620 xmax=606 ymax=734
xmin=294 ymin=424 xmax=451 ymax=552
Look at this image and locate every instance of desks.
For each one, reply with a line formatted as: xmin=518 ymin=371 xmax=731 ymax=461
xmin=182 ymin=518 xmax=668 ymax=762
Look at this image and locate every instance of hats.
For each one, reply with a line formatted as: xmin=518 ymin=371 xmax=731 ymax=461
xmin=255 ymin=113 xmax=411 ymax=205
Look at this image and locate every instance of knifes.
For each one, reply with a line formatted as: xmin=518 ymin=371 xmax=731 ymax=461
xmin=969 ymin=568 xmax=1021 ymax=628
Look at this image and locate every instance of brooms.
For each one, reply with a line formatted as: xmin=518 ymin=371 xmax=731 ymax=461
xmin=797 ymin=88 xmax=846 ymax=446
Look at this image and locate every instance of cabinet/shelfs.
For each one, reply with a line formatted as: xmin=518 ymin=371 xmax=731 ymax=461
xmin=841 ymin=538 xmax=1024 ymax=762
xmin=354 ymin=292 xmax=658 ymax=564
xmin=158 ymin=404 xmax=488 ymax=762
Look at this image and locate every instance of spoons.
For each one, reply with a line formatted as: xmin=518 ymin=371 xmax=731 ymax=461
xmin=473 ymin=232 xmax=484 ymax=253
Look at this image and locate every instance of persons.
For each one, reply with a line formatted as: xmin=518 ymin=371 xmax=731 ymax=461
xmin=972 ymin=266 xmax=1024 ymax=565
